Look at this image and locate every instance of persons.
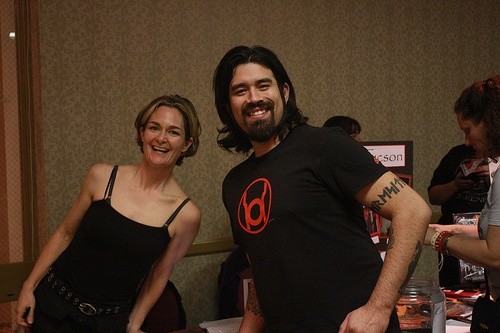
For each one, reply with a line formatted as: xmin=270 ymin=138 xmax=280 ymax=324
xmin=322 ymin=116 xmax=361 ymax=143
xmin=427 ymin=135 xmax=491 ymax=287
xmin=213 ymin=45 xmax=432 ymax=333
xmin=15 ymin=95 xmax=202 ymax=333
xmin=422 ymin=75 xmax=500 ymax=333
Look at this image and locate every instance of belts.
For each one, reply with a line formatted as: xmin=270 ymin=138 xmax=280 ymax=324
xmin=47 ymin=268 xmax=129 ymax=316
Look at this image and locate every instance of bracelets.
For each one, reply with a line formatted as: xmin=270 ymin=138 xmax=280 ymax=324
xmin=430 ymin=231 xmax=453 ymax=256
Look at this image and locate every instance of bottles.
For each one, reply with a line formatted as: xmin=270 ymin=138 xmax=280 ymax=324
xmin=395 ymin=279 xmax=446 ymax=333
